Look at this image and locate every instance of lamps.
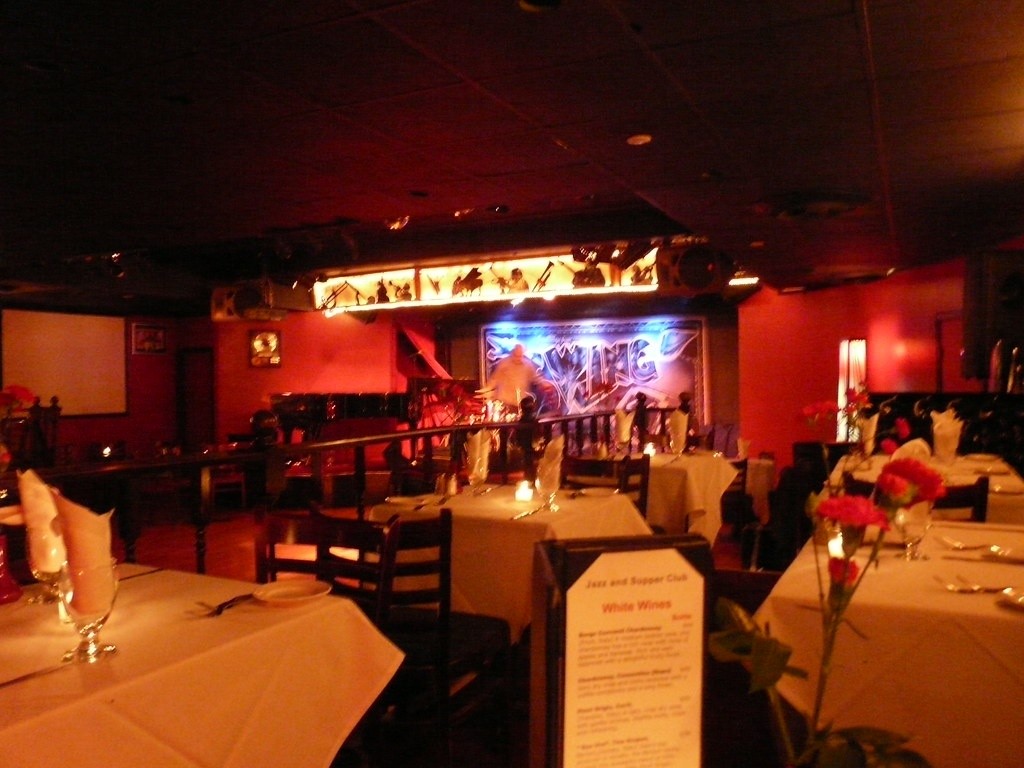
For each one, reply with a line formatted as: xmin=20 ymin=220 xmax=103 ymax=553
xmin=728 ymin=269 xmax=759 ymax=286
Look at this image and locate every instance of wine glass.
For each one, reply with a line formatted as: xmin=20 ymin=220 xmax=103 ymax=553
xmin=534 ymin=473 xmax=560 ymax=512
xmin=25 ymin=527 xmax=70 ymax=604
xmin=57 ymin=557 xmax=119 ymax=665
xmin=893 ymin=502 xmax=932 ymax=561
xmin=616 ymin=434 xmax=629 ymax=458
xmin=465 ymin=464 xmax=490 ymax=496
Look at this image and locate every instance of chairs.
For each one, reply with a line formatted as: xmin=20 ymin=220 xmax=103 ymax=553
xmin=254 ymin=508 xmax=402 ymax=768
xmin=524 ymin=447 xmax=632 ymax=493
xmin=307 ymin=503 xmax=513 ymax=768
xmin=722 ymin=459 xmax=754 ymax=567
xmin=795 ymin=443 xmax=828 ymax=491
xmin=545 ymin=534 xmax=806 ymax=768
xmin=561 ymin=447 xmax=650 ymax=520
xmin=843 ymin=472 xmax=989 ymax=522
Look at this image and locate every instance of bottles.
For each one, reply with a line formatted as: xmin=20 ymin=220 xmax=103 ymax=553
xmin=434 ymin=473 xmax=458 ymax=495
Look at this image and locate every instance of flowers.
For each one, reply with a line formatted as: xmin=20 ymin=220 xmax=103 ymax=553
xmin=797 ymin=402 xmax=900 ymax=527
xmin=844 ymin=387 xmax=911 ymax=460
xmin=707 ymin=456 xmax=947 ymax=768
xmin=0 ymin=381 xmax=37 ymax=477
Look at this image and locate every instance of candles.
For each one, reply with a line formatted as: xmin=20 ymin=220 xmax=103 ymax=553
xmin=644 ymin=444 xmax=656 ymax=457
xmin=515 ymin=480 xmax=533 ymax=501
xmin=828 ymin=532 xmax=845 ymax=560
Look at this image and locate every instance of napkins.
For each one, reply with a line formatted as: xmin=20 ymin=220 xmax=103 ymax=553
xmin=48 ymin=489 xmax=116 ymax=615
xmin=613 ymin=406 xmax=636 ymax=444
xmin=14 ymin=468 xmax=67 ymax=573
xmin=536 ymin=433 xmax=564 ymax=493
xmin=668 ymin=408 xmax=689 ymax=450
xmin=466 ymin=427 xmax=492 ymax=482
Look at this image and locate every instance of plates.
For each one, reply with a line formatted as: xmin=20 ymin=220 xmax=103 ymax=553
xmin=1002 ymin=586 xmax=1024 ymax=609
xmin=253 ymin=579 xmax=333 ymax=608
xmin=386 ymin=496 xmax=414 ymax=505
xmin=991 ymin=544 xmax=1024 ymax=564
xmin=579 ymin=487 xmax=620 ymax=496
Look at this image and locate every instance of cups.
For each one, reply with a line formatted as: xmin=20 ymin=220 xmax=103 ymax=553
xmin=824 ymin=520 xmax=859 ymax=559
xmin=670 ymin=439 xmax=686 ymax=456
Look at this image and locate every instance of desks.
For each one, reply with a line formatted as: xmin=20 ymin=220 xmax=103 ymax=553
xmin=568 ymin=448 xmax=740 ymax=550
xmin=751 ymin=519 xmax=1024 ymax=768
xmin=0 ymin=562 xmax=405 ymax=768
xmin=364 ymin=482 xmax=654 ymax=720
xmin=852 ymin=454 xmax=1024 ymax=525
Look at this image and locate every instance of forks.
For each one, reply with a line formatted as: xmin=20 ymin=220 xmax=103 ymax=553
xmin=932 ymin=573 xmax=1002 ymax=593
xmin=941 ymin=535 xmax=990 ymax=550
xmin=413 ymin=494 xmax=449 ymax=511
xmin=197 ymin=593 xmax=252 ymax=615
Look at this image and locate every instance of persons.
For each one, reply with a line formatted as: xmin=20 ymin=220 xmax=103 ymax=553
xmin=488 ymin=342 xmax=554 ymax=424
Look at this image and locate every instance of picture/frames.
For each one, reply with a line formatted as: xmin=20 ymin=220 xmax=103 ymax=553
xmin=132 ymin=322 xmax=169 ymax=358
